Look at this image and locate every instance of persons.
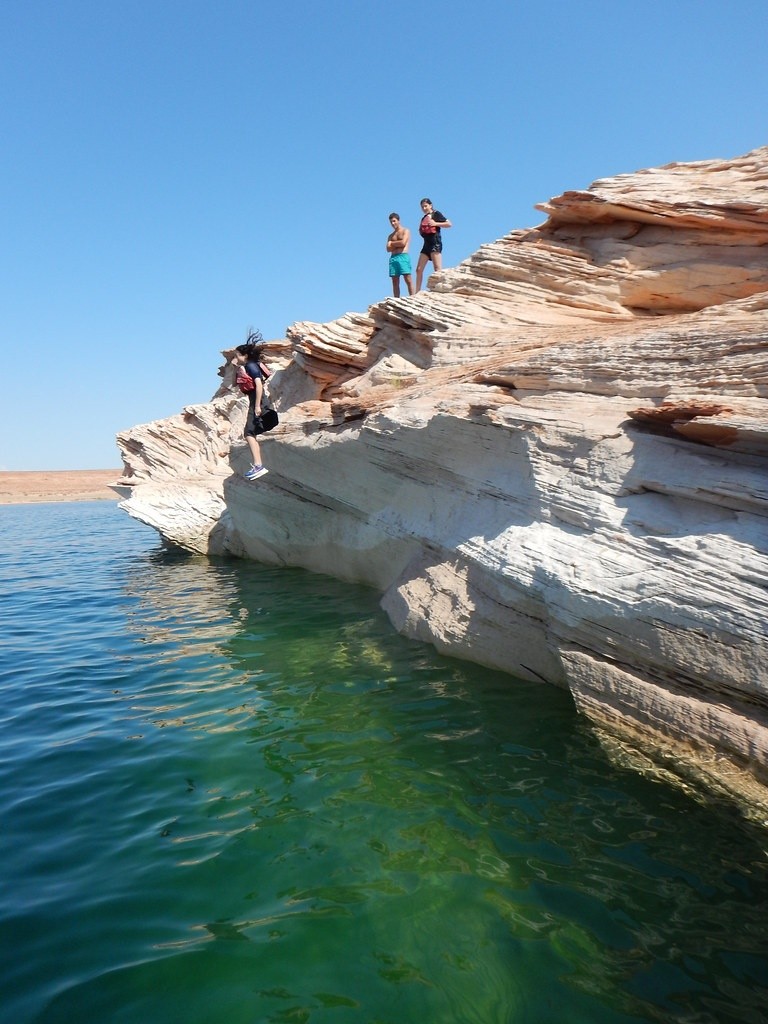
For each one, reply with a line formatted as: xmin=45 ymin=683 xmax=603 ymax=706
xmin=386 ymin=213 xmax=413 ymax=298
xmin=230 ymin=328 xmax=279 ymax=481
xmin=415 ymin=197 xmax=452 ymax=294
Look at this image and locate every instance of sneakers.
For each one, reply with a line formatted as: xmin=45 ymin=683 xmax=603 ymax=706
xmin=245 ymin=462 xmax=265 ymax=477
xmin=249 ymin=469 xmax=268 ymax=481
